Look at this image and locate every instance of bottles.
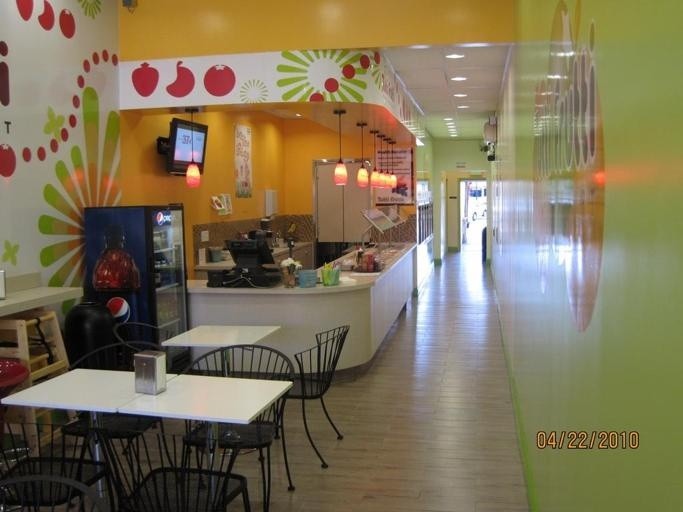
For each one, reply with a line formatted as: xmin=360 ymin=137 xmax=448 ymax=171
xmin=91 ymin=229 xmax=144 ymax=366
xmin=154 ymin=257 xmax=181 ymax=289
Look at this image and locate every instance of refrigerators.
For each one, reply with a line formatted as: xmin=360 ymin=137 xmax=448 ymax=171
xmin=83 ymin=203 xmax=190 ymax=373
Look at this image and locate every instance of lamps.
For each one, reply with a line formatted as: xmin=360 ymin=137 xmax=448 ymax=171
xmin=333 ymin=110 xmax=398 ymax=190
xmin=186 ymin=107 xmax=201 ymax=187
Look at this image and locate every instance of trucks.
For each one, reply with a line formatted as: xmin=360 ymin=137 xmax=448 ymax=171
xmin=468 ymin=185 xmax=486 ymax=220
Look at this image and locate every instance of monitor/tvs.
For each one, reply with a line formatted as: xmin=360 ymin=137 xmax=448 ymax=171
xmin=223 ymin=239 xmax=275 ymax=273
xmin=158 ymin=117 xmax=209 ymax=176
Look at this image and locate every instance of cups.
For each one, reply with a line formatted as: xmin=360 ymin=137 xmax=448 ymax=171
xmin=320 ymin=268 xmax=340 ymax=287
xmin=209 ymin=247 xmax=223 ymax=262
xmin=297 ymin=265 xmax=318 ymax=288
